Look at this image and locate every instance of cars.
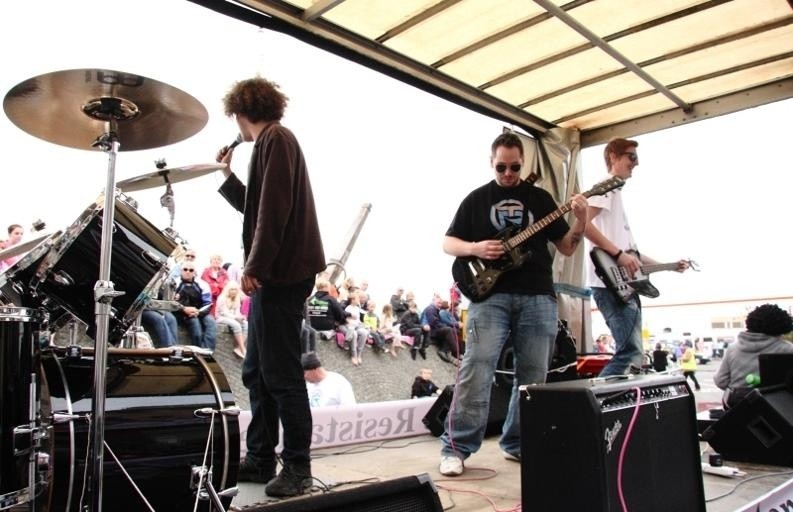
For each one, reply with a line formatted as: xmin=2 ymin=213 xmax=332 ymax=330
xmin=593 ymin=331 xmax=720 ymax=373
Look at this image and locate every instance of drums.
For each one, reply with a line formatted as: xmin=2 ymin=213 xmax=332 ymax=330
xmin=30 ymin=187 xmax=187 ymax=347
xmin=0 ymin=231 xmax=64 ymax=307
xmin=1 ymin=308 xmax=42 ymax=510
xmin=14 ymin=345 xmax=241 ymax=512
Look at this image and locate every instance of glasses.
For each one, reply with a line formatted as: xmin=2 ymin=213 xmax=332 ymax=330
xmin=496 ymin=164 xmax=521 ymax=172
xmin=186 ymin=255 xmax=194 ymax=258
xmin=619 ymin=150 xmax=637 ymax=160
xmin=184 ymin=268 xmax=194 ymax=272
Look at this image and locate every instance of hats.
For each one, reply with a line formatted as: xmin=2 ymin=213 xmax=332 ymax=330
xmin=302 ymin=353 xmax=321 ymax=369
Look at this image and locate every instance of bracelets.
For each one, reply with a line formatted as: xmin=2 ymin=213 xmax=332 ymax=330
xmin=613 ymin=248 xmax=623 ymax=260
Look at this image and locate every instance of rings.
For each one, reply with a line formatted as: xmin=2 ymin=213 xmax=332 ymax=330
xmin=578 ymin=200 xmax=583 ymax=205
xmin=574 ymin=203 xmax=580 ymax=208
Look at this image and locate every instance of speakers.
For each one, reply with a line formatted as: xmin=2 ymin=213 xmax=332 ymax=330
xmin=518 ymin=375 xmax=706 ymax=512
xmin=703 ymin=383 xmax=793 ymax=467
xmin=243 ymin=472 xmax=444 ymax=512
xmin=422 ymin=383 xmax=510 ymax=437
xmin=759 ymin=353 xmax=793 ymax=387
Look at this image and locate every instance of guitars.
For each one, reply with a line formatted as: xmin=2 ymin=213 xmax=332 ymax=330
xmin=452 ymin=176 xmax=624 ymax=303
xmin=588 ymin=247 xmax=699 ymax=306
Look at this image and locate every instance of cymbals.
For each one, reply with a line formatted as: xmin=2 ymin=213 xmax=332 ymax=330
xmin=116 ymin=164 xmax=226 ymax=192
xmin=2 ymin=69 xmax=209 ymax=152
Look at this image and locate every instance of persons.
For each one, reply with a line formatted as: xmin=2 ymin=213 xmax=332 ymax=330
xmin=215 ymin=73 xmax=326 ymax=494
xmin=300 ymin=276 xmax=466 ymax=365
xmin=438 ymin=131 xmax=590 ymax=477
xmin=140 ymin=245 xmax=251 ymax=359
xmin=711 ymin=303 xmax=793 ymax=411
xmin=583 ymin=138 xmax=689 ymax=383
xmin=301 ymin=351 xmax=356 ymax=406
xmin=0 ymin=224 xmax=26 ymax=271
xmin=592 ymin=335 xmax=615 ymax=355
xmin=644 ymin=337 xmax=728 ymax=391
xmin=411 ymin=368 xmax=442 ymax=400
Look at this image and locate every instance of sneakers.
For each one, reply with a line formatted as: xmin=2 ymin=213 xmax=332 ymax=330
xmin=440 ymin=454 xmax=464 ymax=476
xmin=240 ymin=457 xmax=277 ymax=483
xmin=499 ymin=448 xmax=522 ymax=462
xmin=350 ymin=354 xmax=361 ymax=364
xmin=419 ymin=348 xmax=425 ymax=359
xmin=266 ymin=461 xmax=313 ymax=497
xmin=411 ymin=349 xmax=417 ymax=359
xmin=438 ymin=351 xmax=450 ymax=363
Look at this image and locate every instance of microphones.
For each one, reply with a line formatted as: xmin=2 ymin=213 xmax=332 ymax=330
xmin=223 ymin=134 xmax=242 ymax=156
